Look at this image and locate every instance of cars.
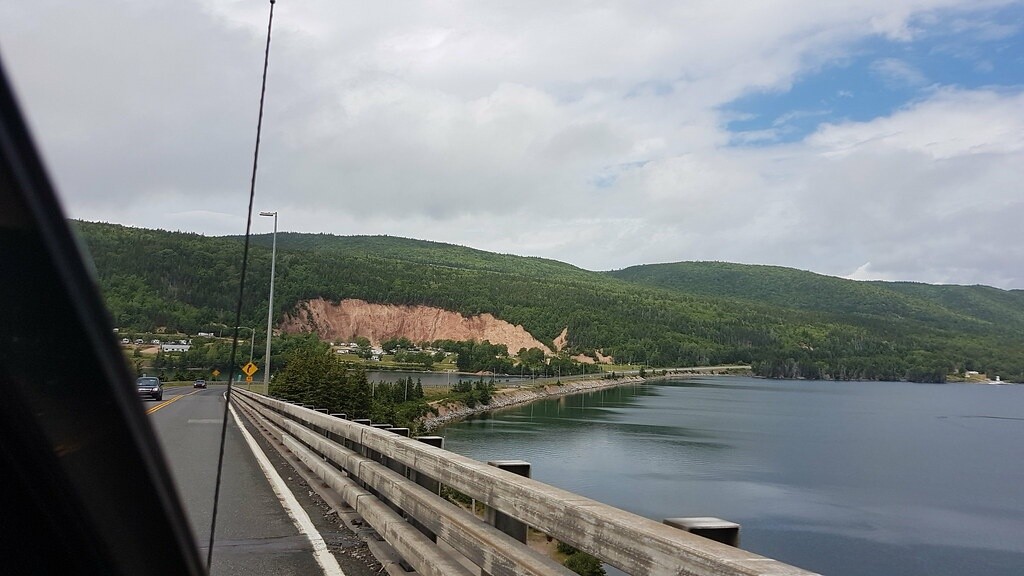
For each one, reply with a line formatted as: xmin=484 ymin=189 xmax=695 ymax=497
xmin=193 ymin=380 xmax=208 ymax=389
xmin=135 ymin=376 xmax=165 ymax=402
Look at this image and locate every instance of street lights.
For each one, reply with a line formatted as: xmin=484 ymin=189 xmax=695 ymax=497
xmin=555 ymin=365 xmax=560 ymax=381
xmin=371 ymin=380 xmax=375 ymax=396
xmin=258 ymin=211 xmax=278 ymax=397
xmin=521 ymin=365 xmax=525 ymax=381
xmin=234 ymin=326 xmax=255 ymax=391
xmin=532 ymin=367 xmax=534 ymax=386
xmin=405 ymin=376 xmax=408 ymax=401
xmin=447 ymin=370 xmax=451 ymax=394
